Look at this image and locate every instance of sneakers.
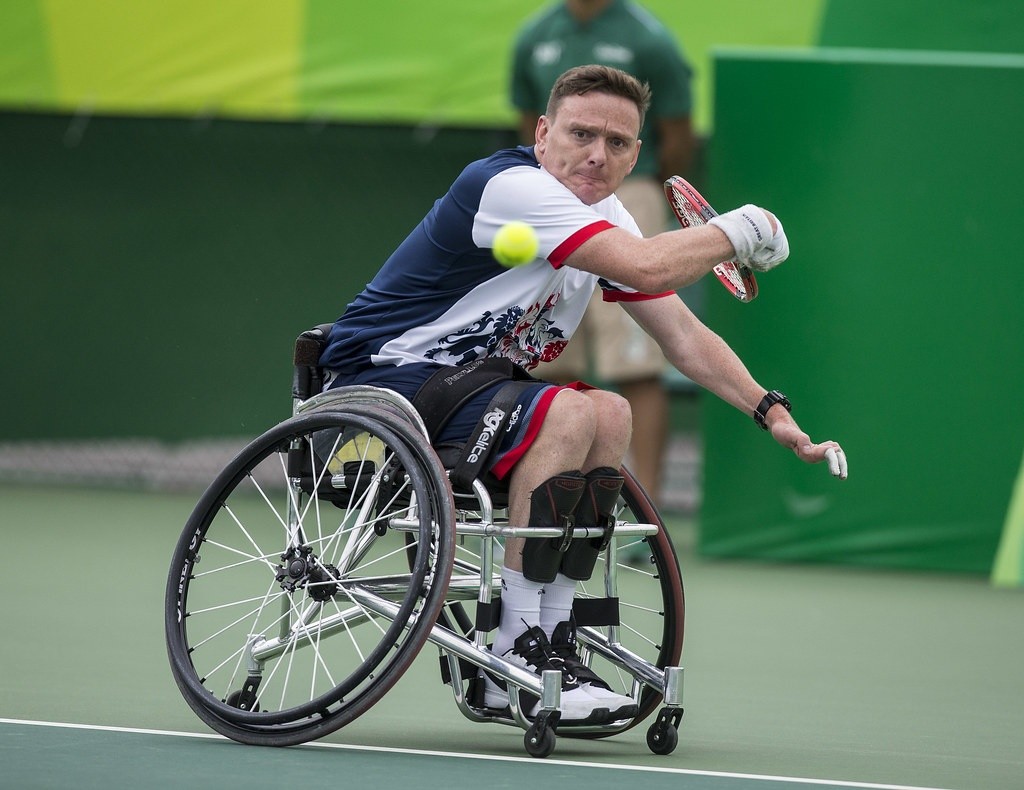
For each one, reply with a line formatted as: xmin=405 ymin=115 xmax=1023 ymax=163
xmin=476 ymin=618 xmax=638 ymax=725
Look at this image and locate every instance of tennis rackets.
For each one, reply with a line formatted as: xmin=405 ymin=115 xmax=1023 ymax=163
xmin=662 ymin=174 xmax=758 ymax=303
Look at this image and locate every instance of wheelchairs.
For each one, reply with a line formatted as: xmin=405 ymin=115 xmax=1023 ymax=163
xmin=162 ymin=325 xmax=687 ymax=763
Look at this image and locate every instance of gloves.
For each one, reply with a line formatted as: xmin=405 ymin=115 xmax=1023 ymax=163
xmin=749 ymin=207 xmax=790 ymax=271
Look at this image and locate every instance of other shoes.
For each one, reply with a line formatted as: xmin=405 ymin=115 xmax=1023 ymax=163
xmin=616 ymin=507 xmax=651 ymax=564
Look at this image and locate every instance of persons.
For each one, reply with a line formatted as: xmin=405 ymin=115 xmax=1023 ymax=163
xmin=506 ymin=2 xmax=699 ymax=560
xmin=294 ymin=63 xmax=852 ymax=728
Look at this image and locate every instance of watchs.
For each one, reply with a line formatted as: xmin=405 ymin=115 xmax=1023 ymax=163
xmin=753 ymin=389 xmax=793 ymax=432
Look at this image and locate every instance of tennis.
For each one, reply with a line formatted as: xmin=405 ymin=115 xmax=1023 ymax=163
xmin=489 ymin=218 xmax=540 ymax=267
